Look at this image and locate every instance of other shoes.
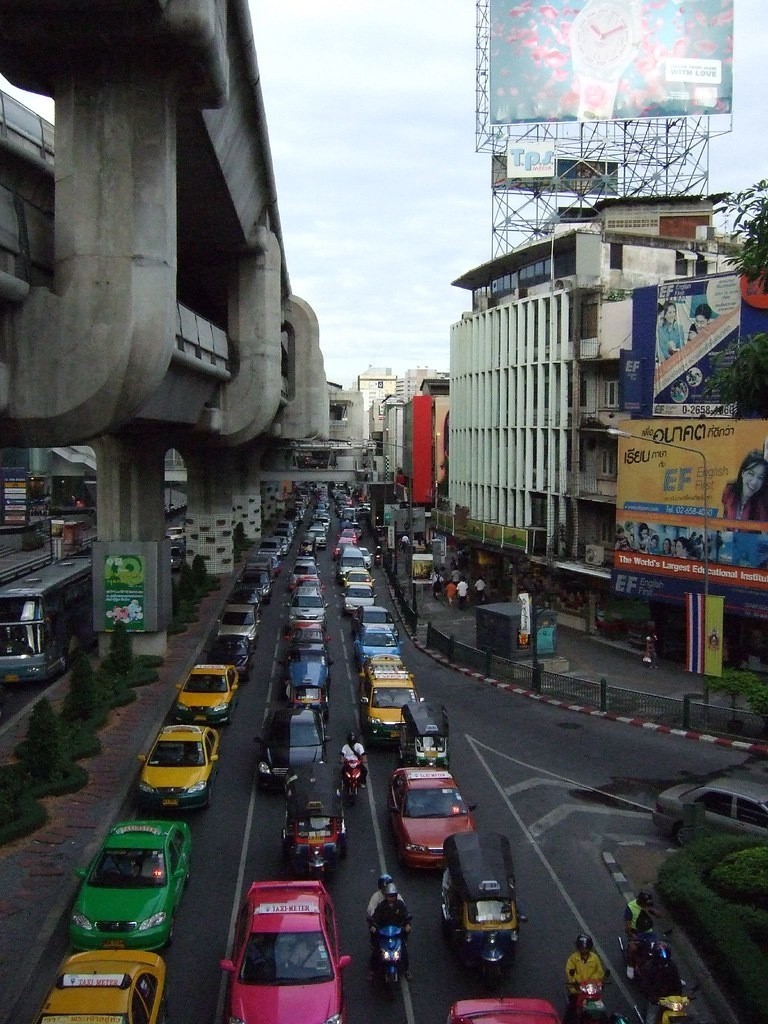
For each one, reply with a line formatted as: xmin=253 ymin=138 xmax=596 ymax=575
xmin=367 ymin=968 xmax=375 ymax=980
xmin=627 ymin=966 xmax=635 ymax=979
xmin=361 ymin=783 xmax=366 ymax=789
xmin=403 ymin=968 xmax=413 ymax=983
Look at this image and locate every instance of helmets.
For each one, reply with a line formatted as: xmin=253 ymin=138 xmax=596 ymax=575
xmin=347 ymin=731 xmax=357 ymax=743
xmin=638 ymin=890 xmax=653 ymax=905
xmin=383 ymin=883 xmax=398 ymax=894
xmin=378 ymin=874 xmax=393 ymax=889
xmin=576 ymin=934 xmax=593 ymax=949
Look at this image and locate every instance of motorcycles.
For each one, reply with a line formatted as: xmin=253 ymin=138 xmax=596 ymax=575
xmin=283 ymin=760 xmax=349 ymax=881
xmin=440 ymin=832 xmax=529 ymax=996
xmin=337 ymin=751 xmax=369 ymax=806
xmin=287 ymin=662 xmax=331 ymax=725
xmin=401 ymin=701 xmax=451 ymax=768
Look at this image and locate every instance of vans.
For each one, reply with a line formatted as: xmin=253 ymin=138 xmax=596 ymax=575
xmin=358 ymin=653 xmax=426 ymax=752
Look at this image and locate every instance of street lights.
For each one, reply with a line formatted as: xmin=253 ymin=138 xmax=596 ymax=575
xmin=606 ymin=428 xmax=709 ymax=596
xmin=365 ymin=439 xmax=414 ymax=554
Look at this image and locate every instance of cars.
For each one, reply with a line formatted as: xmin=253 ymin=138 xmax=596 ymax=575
xmin=203 ymin=634 xmax=255 ymax=680
xmin=32 ymin=951 xmax=170 ymax=1024
xmin=252 ymin=707 xmax=331 ymax=793
xmin=218 ymin=879 xmax=352 ymax=1024
xmin=67 ymin=820 xmax=194 ymax=953
xmin=135 ymin=723 xmax=221 ymax=817
xmin=210 ymin=481 xmax=404 ymax=692
xmin=388 ymin=766 xmax=478 ymax=872
xmin=164 ymin=527 xmax=187 ymax=572
xmin=173 ymin=663 xmax=241 ymax=730
xmin=653 ymin=777 xmax=768 ymax=847
xmin=447 ymin=996 xmax=564 ymax=1024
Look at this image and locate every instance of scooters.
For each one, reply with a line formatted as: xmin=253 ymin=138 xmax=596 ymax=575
xmin=367 ymin=916 xmax=414 ymax=997
xmin=618 ymin=924 xmax=700 ymax=1024
xmin=565 ymin=968 xmax=612 ymax=1024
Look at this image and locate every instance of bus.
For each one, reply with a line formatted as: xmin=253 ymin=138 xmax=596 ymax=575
xmin=1 ymin=555 xmax=94 ymax=684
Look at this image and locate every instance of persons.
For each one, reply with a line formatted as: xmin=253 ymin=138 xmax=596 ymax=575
xmin=430 ymin=567 xmax=469 ymax=610
xmin=688 ymin=303 xmax=711 ymax=341
xmin=404 ymin=518 xmax=410 ymax=532
xmin=645 ymin=631 xmax=658 ymax=669
xmin=474 ymin=575 xmax=486 ymax=604
xmin=623 ymin=889 xmax=665 ymax=980
xmin=721 ymin=453 xmax=768 ymax=534
xmin=654 ymin=302 xmax=685 ymax=367
xmin=641 ymin=944 xmax=680 ymax=1024
xmin=373 ymin=546 xmax=382 ymax=565
xmin=401 ymin=535 xmax=409 ymax=553
xmin=616 ymin=522 xmax=723 ymax=562
xmin=366 ymin=873 xmax=412 ymax=949
xmin=336 ymin=732 xmax=368 ymax=796
xmin=563 ymin=934 xmax=604 ymax=1024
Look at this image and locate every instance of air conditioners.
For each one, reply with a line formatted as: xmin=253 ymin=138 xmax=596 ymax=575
xmin=553 ymin=275 xmax=579 ymax=290
xmin=585 ymin=545 xmax=605 ymax=565
